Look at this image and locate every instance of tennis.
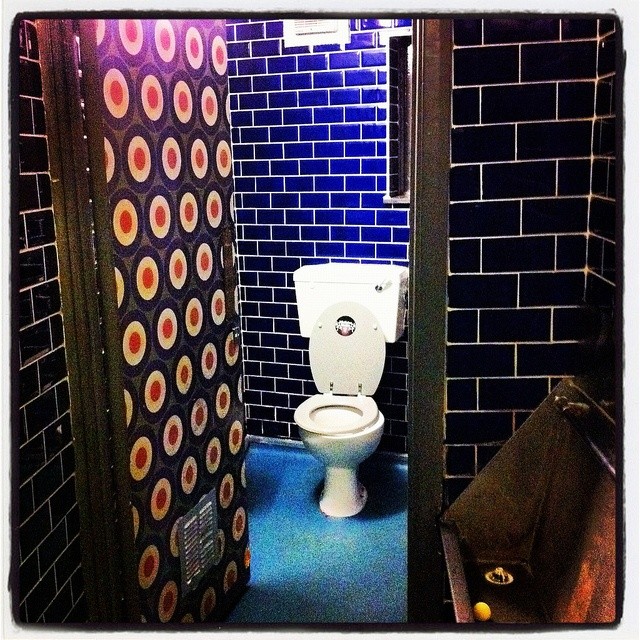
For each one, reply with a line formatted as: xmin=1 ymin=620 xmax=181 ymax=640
xmin=472 ymin=601 xmax=492 ymax=622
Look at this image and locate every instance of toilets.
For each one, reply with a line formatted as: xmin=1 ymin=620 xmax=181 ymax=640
xmin=292 ymin=257 xmax=410 ymax=520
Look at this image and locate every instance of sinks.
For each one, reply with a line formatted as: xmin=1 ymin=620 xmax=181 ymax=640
xmin=436 ymin=375 xmax=622 ymax=625
